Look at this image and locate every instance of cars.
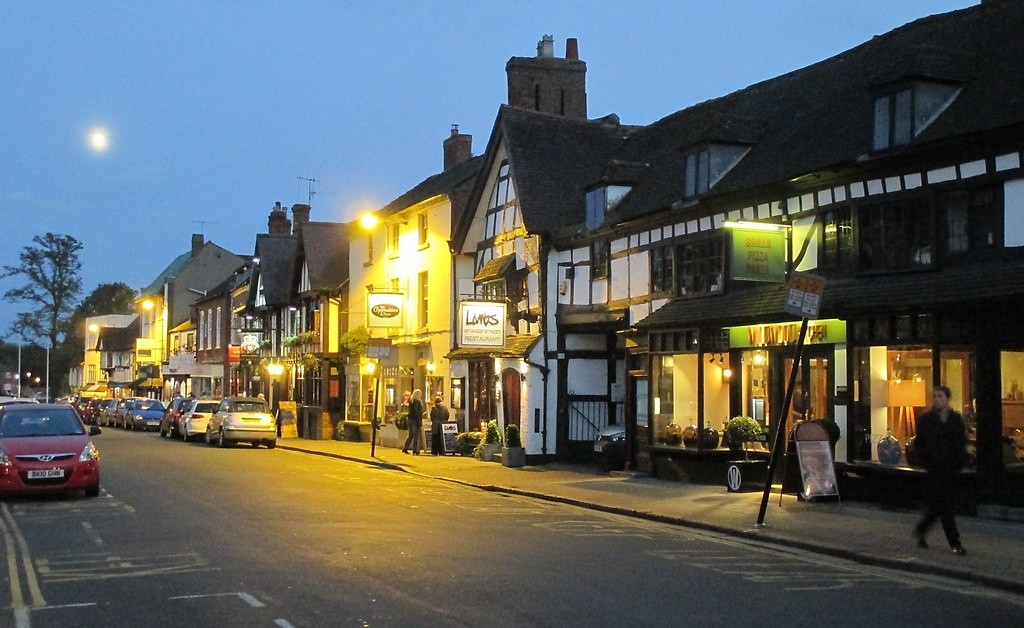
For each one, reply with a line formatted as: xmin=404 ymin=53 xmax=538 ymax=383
xmin=160 ymin=398 xmax=191 ymax=439
xmin=178 ymin=400 xmax=221 ymax=442
xmin=75 ymin=397 xmax=167 ymax=431
xmin=205 ymin=398 xmax=277 ymax=449
xmin=0 ymin=398 xmax=40 ymax=405
xmin=0 ymin=404 xmax=100 ymax=497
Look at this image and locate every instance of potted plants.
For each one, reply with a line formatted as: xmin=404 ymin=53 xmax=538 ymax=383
xmin=479 ymin=421 xmax=501 ymax=460
xmin=723 ymin=416 xmax=767 ymax=493
xmin=501 ymin=424 xmax=526 ymax=468
xmin=456 ymin=431 xmax=483 ymax=456
xmin=396 ymin=412 xmax=414 ymax=451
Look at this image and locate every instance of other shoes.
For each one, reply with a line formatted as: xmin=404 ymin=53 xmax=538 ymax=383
xmin=413 ymin=450 xmax=419 ymax=455
xmin=912 ymin=532 xmax=929 ymax=548
xmin=402 ymin=449 xmax=410 ymax=454
xmin=951 ymin=546 xmax=966 ymax=555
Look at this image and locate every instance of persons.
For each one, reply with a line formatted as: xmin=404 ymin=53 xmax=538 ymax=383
xmin=173 ymin=390 xmax=209 ymax=399
xmin=402 ymin=389 xmax=424 ymax=455
xmin=402 ymin=390 xmax=412 ymax=412
xmin=430 ymin=396 xmax=448 ymax=456
xmin=910 ymin=384 xmax=967 ymax=554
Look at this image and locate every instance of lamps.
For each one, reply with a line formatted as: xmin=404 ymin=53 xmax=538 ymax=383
xmin=709 ymin=353 xmax=715 ymax=363
xmin=718 ymin=353 xmax=724 ymax=364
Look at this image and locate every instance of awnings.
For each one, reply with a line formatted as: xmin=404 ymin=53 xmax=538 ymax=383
xmin=442 ymin=333 xmax=550 ymax=371
xmin=128 ymin=377 xmax=163 ymax=389
